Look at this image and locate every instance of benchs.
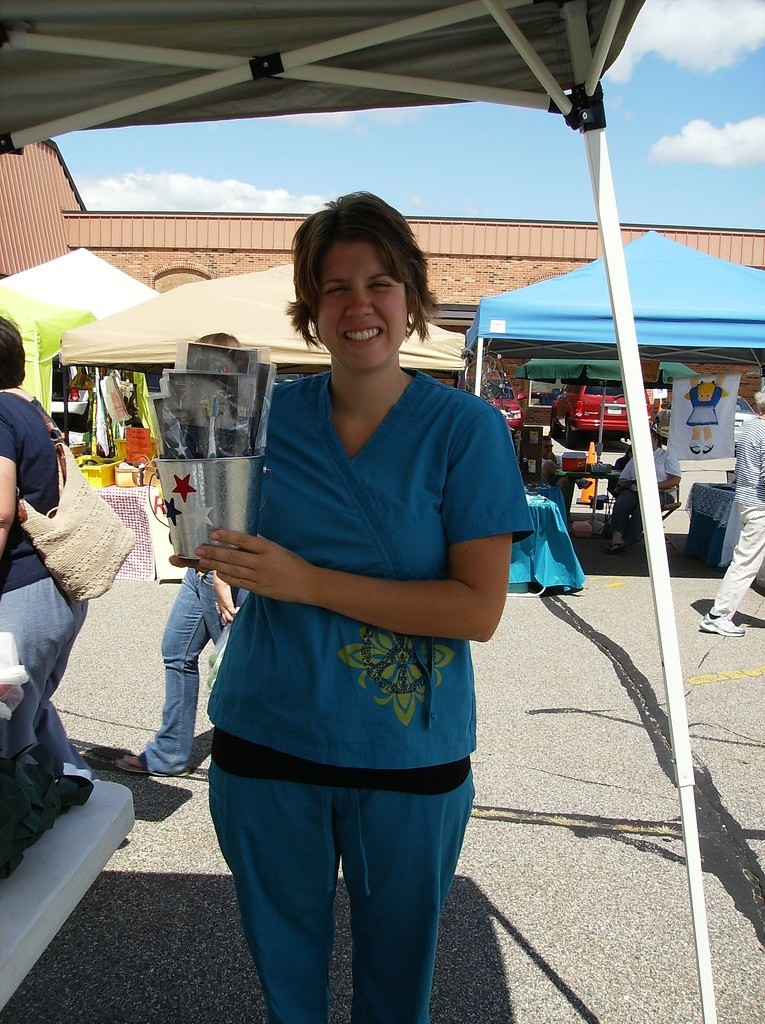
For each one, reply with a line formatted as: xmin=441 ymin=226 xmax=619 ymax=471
xmin=0 ymin=781 xmax=137 ymax=1013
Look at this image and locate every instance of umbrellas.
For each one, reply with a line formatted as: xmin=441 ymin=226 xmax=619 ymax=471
xmin=511 ymin=358 xmax=702 ymax=538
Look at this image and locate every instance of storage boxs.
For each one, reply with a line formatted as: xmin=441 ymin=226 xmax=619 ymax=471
xmin=73 ymin=454 xmax=125 ymax=489
xmin=113 ymin=464 xmax=141 ymax=487
xmin=562 ymin=451 xmax=586 ymax=472
xmin=115 ymin=438 xmax=158 ymax=464
xmin=142 ymin=466 xmax=161 ymax=485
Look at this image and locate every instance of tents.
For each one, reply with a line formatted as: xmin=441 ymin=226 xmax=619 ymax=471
xmin=0 ymin=247 xmax=162 ymax=462
xmin=63 ymin=261 xmax=467 ymax=450
xmin=463 ymin=230 xmax=765 ymax=401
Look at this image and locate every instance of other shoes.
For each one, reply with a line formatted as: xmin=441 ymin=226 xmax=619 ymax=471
xmin=567 ymin=515 xmax=573 ymax=523
xmin=577 ymin=479 xmax=593 ymax=489
xmin=608 ymin=540 xmax=624 ymax=551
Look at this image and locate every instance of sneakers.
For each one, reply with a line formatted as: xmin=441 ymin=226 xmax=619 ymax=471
xmin=700 ymin=613 xmax=745 ymax=637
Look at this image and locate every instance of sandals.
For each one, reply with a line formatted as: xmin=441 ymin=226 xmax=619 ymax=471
xmin=116 ymin=754 xmax=190 ymax=777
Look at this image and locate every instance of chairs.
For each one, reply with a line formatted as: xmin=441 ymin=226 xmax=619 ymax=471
xmin=632 ymin=484 xmax=682 ymax=548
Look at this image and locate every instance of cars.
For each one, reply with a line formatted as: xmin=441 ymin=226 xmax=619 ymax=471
xmin=551 ymin=378 xmax=656 ymax=450
xmin=455 ymin=372 xmax=528 ymax=436
xmin=652 ymin=392 xmax=760 ymax=454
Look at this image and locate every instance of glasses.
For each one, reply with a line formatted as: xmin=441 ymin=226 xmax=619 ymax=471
xmin=545 ymin=444 xmax=554 ymax=448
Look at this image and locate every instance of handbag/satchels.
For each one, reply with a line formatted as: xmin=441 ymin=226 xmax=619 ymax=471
xmin=207 ymin=623 xmax=232 ymax=695
xmin=0 ymin=743 xmax=95 ymax=878
xmin=0 ymin=388 xmax=136 ymax=604
xmin=69 ymin=368 xmax=94 ymax=390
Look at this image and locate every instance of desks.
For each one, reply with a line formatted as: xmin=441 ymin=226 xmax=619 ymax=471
xmin=94 ymin=484 xmax=188 ymax=582
xmin=684 ymin=482 xmax=745 ymax=572
xmin=506 ymin=470 xmax=623 ymax=596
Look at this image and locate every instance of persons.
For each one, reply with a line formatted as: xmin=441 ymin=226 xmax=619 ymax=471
xmin=168 ymin=190 xmax=536 ymax=1024
xmin=606 ymin=429 xmax=681 ymax=555
xmin=117 ymin=333 xmax=251 ymax=774
xmin=700 ymin=388 xmax=765 ymax=637
xmin=541 ymin=434 xmax=575 ymax=524
xmin=0 ymin=315 xmax=99 ymax=782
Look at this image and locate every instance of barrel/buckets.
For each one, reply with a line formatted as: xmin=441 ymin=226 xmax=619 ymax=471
xmin=148 ymin=453 xmax=271 ymax=559
